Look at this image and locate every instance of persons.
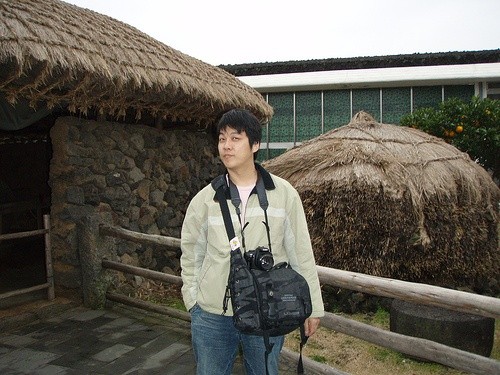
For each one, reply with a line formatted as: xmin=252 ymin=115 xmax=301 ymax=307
xmin=179 ymin=109 xmax=324 ymax=375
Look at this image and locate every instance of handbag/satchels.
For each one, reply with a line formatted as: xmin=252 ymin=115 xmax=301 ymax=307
xmin=228 ymin=250 xmax=312 ymax=337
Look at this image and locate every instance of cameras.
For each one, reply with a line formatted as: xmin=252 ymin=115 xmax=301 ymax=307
xmin=244 ymin=245 xmax=274 ymax=271
